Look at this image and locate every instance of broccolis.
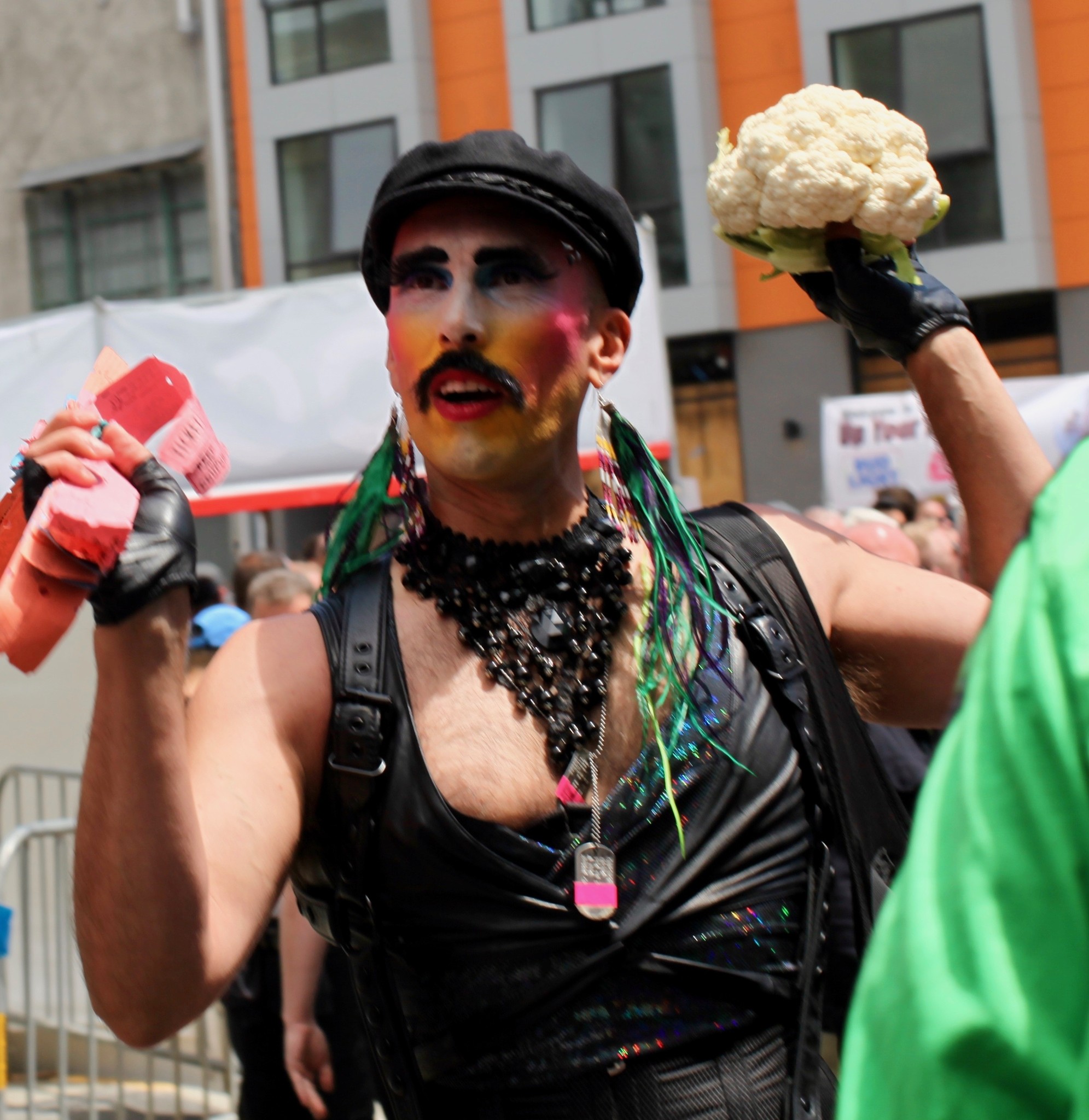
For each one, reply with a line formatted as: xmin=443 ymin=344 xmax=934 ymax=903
xmin=707 ymin=83 xmax=948 ymax=275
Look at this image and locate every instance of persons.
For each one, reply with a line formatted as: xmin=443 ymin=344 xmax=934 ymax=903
xmin=756 ymin=489 xmax=977 ymax=822
xmin=837 ymin=436 xmax=1089 ymax=1120
xmin=22 ymin=129 xmax=1052 ymax=1120
xmin=184 ymin=531 xmax=375 ymax=1120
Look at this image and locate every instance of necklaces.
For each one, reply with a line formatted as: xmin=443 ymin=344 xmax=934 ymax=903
xmin=392 ymin=479 xmax=634 ymax=921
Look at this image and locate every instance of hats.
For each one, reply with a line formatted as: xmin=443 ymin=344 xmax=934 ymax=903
xmin=873 ymin=488 xmax=917 ymax=521
xmin=360 ymin=128 xmax=644 ymax=319
